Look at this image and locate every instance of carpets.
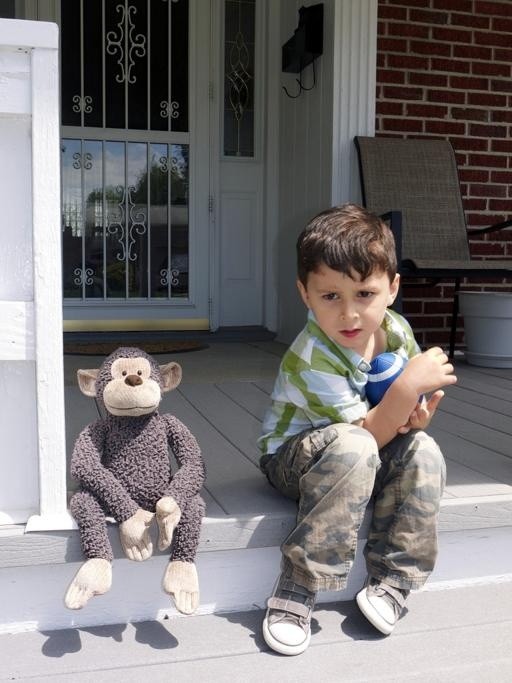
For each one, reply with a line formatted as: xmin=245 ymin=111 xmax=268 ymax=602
xmin=65 ymin=337 xmax=289 ymax=388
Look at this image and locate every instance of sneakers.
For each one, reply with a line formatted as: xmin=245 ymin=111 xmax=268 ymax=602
xmin=356 ymin=574 xmax=412 ymax=635
xmin=262 ymin=572 xmax=318 ymax=656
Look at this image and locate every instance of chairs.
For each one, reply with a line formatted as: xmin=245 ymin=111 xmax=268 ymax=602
xmin=353 ymin=135 xmax=511 ymax=359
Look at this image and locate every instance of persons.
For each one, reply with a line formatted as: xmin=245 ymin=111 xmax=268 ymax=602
xmin=258 ymin=199 xmax=459 ymax=658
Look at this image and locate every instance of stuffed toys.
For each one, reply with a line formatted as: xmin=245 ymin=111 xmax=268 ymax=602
xmin=61 ymin=346 xmax=207 ymax=616
